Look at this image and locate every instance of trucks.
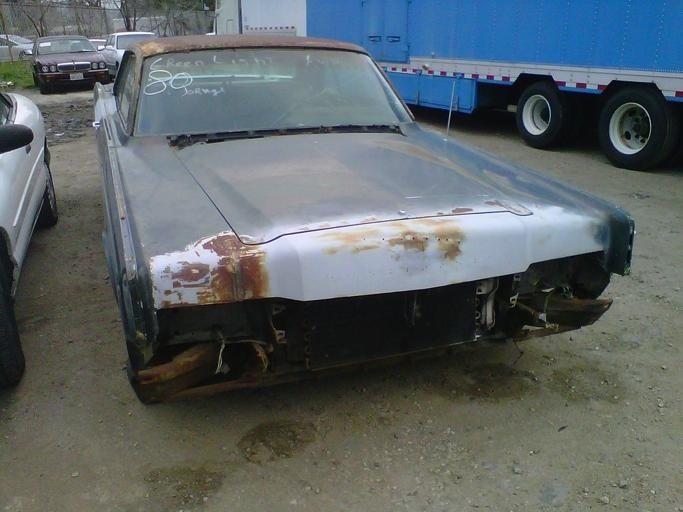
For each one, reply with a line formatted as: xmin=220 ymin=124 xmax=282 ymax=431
xmin=206 ymin=0 xmax=683 ymax=170
